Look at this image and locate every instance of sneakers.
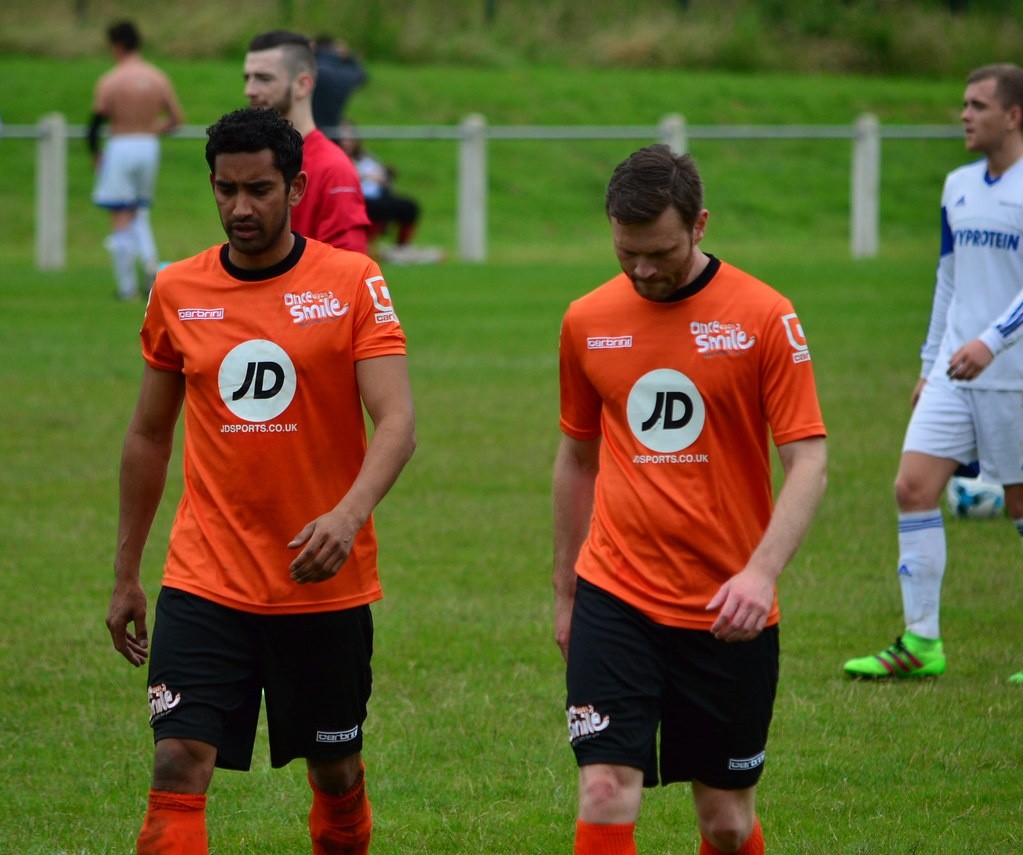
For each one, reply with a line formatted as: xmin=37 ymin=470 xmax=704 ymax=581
xmin=1006 ymin=671 xmax=1023 ymax=685
xmin=843 ymin=636 xmax=946 ymax=678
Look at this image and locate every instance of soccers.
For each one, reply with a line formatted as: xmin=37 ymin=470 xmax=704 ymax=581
xmin=946 ymin=475 xmax=1005 ymax=520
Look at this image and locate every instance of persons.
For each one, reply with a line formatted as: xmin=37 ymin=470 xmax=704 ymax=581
xmin=550 ymin=144 xmax=828 ymax=855
xmin=309 ymin=36 xmax=367 ymax=126
xmin=243 ymin=31 xmax=371 ymax=257
xmin=842 ymin=63 xmax=1023 ymax=685
xmin=338 ymin=120 xmax=420 ymax=250
xmin=103 ymin=109 xmax=419 ymax=855
xmin=84 ymin=21 xmax=184 ymax=304
